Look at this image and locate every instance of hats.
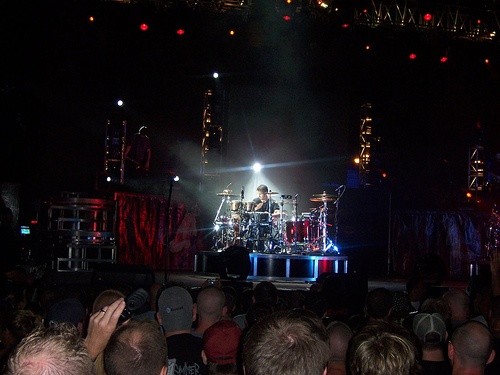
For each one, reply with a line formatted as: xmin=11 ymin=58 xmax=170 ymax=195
xmin=411 ymin=307 xmax=447 ymax=345
xmin=49 ymin=294 xmax=82 ymax=324
xmin=158 ymin=285 xmax=192 ymax=330
xmin=203 ymin=319 xmax=244 ymax=363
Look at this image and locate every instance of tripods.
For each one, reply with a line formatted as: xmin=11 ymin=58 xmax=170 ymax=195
xmin=308 ymin=201 xmax=341 ymax=255
xmin=211 ymin=226 xmax=228 ymax=251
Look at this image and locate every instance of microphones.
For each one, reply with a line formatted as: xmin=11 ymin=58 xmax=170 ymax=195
xmin=336 ymin=185 xmax=344 ymax=192
xmin=241 ymin=187 xmax=244 ymax=199
xmin=289 ymin=194 xmax=299 ymax=203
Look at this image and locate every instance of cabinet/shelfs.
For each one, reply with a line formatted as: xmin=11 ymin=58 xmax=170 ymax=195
xmin=47 ymin=196 xmax=117 ymax=273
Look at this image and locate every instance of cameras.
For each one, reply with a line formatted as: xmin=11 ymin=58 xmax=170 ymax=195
xmin=118 ymin=308 xmax=133 ymax=322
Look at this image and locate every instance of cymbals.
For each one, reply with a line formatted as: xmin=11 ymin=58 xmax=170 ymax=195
xmin=261 ymin=192 xmax=278 ymax=194
xmin=309 ymin=194 xmax=338 ymax=202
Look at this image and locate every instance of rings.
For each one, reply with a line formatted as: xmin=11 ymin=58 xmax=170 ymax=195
xmin=101 ymin=308 xmax=106 ymax=313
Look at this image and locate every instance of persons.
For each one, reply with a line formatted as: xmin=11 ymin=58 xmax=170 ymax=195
xmin=246 ymin=184 xmax=279 ymax=253
xmin=0 ymin=256 xmax=500 ymax=375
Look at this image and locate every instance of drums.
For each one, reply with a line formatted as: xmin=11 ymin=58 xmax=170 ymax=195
xmin=285 ymin=212 xmax=318 ymax=245
xmin=213 ymin=200 xmax=272 ymax=251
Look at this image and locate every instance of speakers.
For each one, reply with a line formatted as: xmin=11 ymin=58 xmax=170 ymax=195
xmin=90 ymin=261 xmax=155 ymax=292
